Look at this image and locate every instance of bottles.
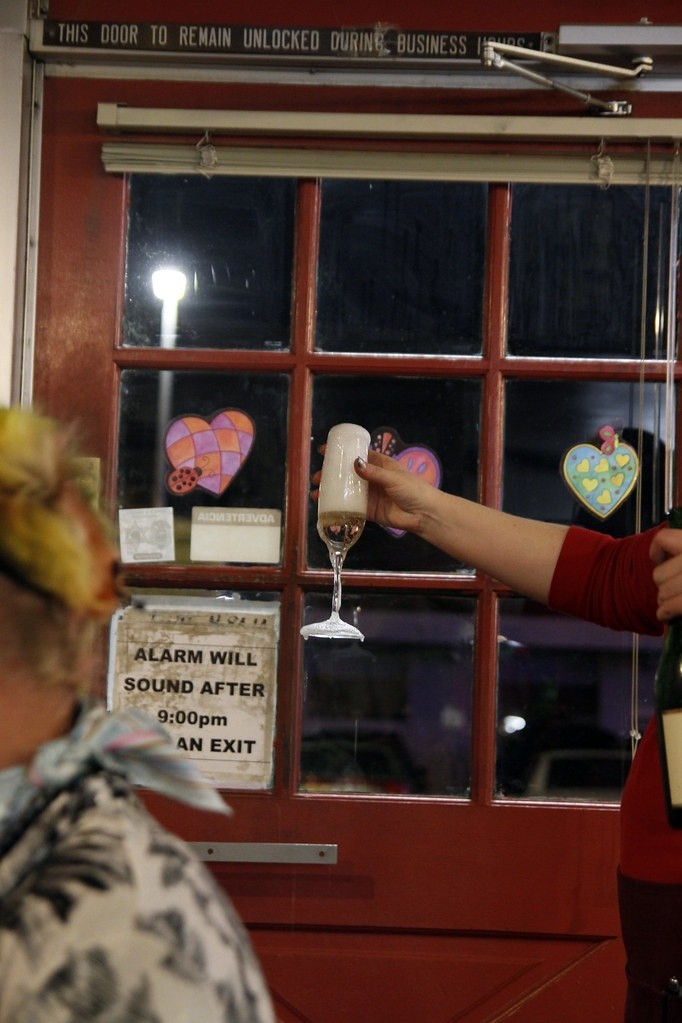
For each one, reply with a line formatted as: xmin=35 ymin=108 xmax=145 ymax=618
xmin=652 ymin=507 xmax=682 ymax=830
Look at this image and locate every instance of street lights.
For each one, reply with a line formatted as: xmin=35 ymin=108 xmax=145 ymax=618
xmin=149 ymin=264 xmax=196 ymax=508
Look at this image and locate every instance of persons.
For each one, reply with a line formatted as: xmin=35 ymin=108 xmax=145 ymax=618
xmin=0 ymin=406 xmax=281 ymax=1022
xmin=309 ymin=442 xmax=682 ymax=1023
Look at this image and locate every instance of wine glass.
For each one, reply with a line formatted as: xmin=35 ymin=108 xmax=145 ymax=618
xmin=298 ymin=423 xmax=370 ymax=640
